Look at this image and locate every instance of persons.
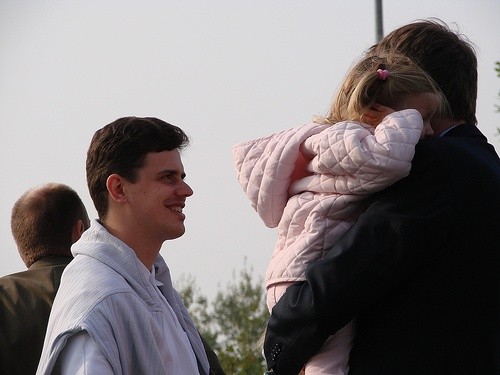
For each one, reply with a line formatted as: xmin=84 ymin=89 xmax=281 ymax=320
xmin=1 ymin=181 xmax=227 ymax=374
xmin=230 ymin=48 xmax=453 ymax=375
xmin=263 ymin=18 xmax=499 ymax=375
xmin=33 ymin=115 xmax=213 ymax=375
